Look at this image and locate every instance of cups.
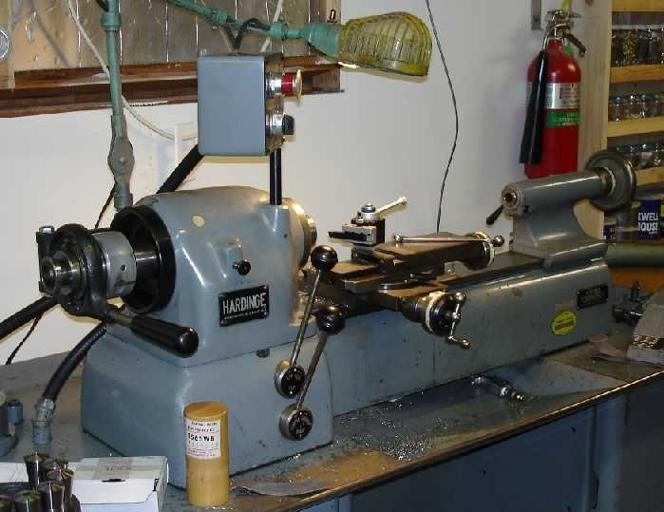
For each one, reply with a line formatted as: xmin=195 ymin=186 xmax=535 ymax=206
xmin=610 ymin=91 xmax=664 ymax=120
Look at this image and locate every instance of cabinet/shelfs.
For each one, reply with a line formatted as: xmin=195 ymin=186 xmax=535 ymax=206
xmin=569 ymin=0 xmax=663 ymax=239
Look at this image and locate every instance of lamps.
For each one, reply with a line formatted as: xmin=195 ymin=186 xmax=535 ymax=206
xmin=103 ymin=0 xmax=432 ymax=210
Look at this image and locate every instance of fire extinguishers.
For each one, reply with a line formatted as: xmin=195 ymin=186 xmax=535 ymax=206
xmin=519 ymin=10 xmax=588 ymax=180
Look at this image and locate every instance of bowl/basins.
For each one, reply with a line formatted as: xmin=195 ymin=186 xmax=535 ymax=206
xmin=617 ymin=142 xmax=664 ymax=169
